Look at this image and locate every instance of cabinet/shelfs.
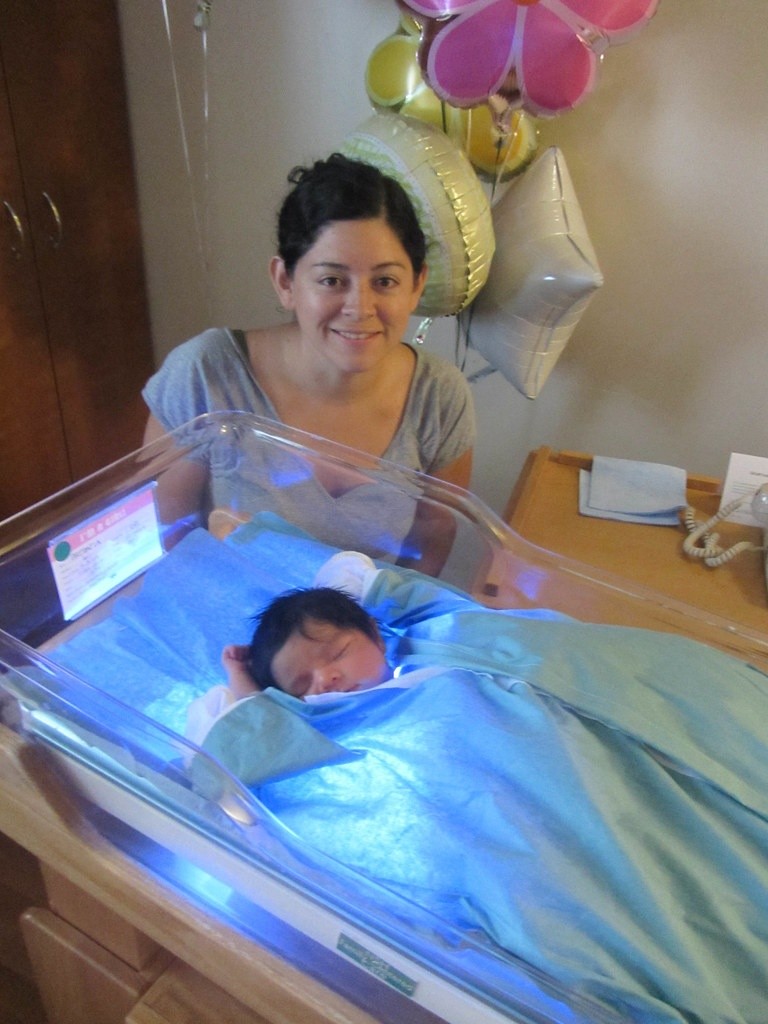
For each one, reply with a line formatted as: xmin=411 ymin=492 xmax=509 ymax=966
xmin=0 ymin=0 xmax=165 ymax=553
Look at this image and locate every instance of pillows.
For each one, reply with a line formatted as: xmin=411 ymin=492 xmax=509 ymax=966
xmin=185 ymin=550 xmax=383 ymax=825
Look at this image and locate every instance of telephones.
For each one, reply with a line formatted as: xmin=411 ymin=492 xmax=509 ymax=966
xmin=751 ymin=483 xmax=768 ymax=521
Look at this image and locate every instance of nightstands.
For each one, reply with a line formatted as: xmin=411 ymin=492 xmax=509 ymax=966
xmin=470 ymin=445 xmax=768 ymax=674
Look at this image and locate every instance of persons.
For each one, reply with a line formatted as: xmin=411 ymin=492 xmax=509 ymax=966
xmin=140 ymin=151 xmax=474 ymax=579
xmin=220 ymin=586 xmax=395 ymax=702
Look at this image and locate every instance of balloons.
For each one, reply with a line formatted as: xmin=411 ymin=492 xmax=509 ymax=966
xmin=333 ymin=1 xmax=657 ymax=398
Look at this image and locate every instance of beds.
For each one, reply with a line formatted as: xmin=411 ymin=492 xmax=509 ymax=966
xmin=0 ymin=409 xmax=768 ymax=1022
